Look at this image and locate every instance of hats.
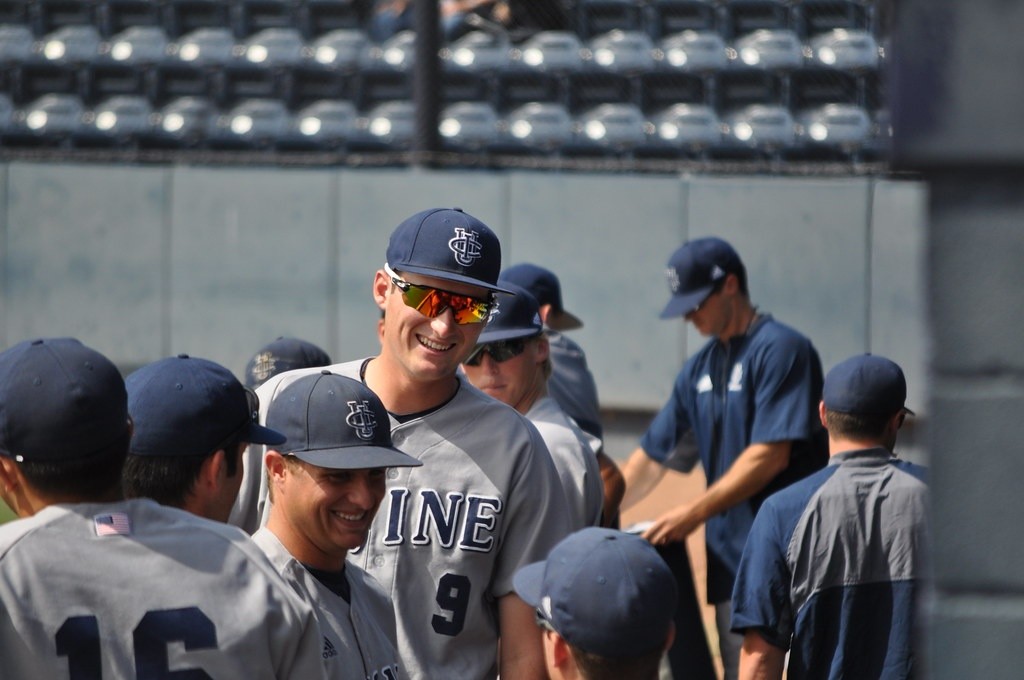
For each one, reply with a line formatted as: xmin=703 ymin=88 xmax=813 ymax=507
xmin=823 ymin=352 xmax=916 ymax=417
xmin=660 ymin=238 xmax=745 ymax=317
xmin=125 ymin=354 xmax=287 ymax=460
xmin=0 ymin=339 xmax=128 ymax=464
xmin=511 ymin=526 xmax=675 ymax=657
xmin=475 ymin=281 xmax=542 ymax=343
xmin=498 ymin=263 xmax=583 ymax=330
xmin=266 ymin=371 xmax=423 ymax=470
xmin=386 ymin=207 xmax=513 ymax=295
xmin=246 ymin=337 xmax=331 ymax=391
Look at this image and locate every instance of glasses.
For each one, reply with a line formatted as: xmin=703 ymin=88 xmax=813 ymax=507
xmin=461 ymin=338 xmax=523 ymax=366
xmin=385 ymin=262 xmax=496 ymax=326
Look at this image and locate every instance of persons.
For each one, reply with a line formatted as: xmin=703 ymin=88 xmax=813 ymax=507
xmin=0 ymin=336 xmax=424 ymax=680
xmin=459 ymin=237 xmax=928 ymax=680
xmin=225 ymin=208 xmax=569 ymax=680
